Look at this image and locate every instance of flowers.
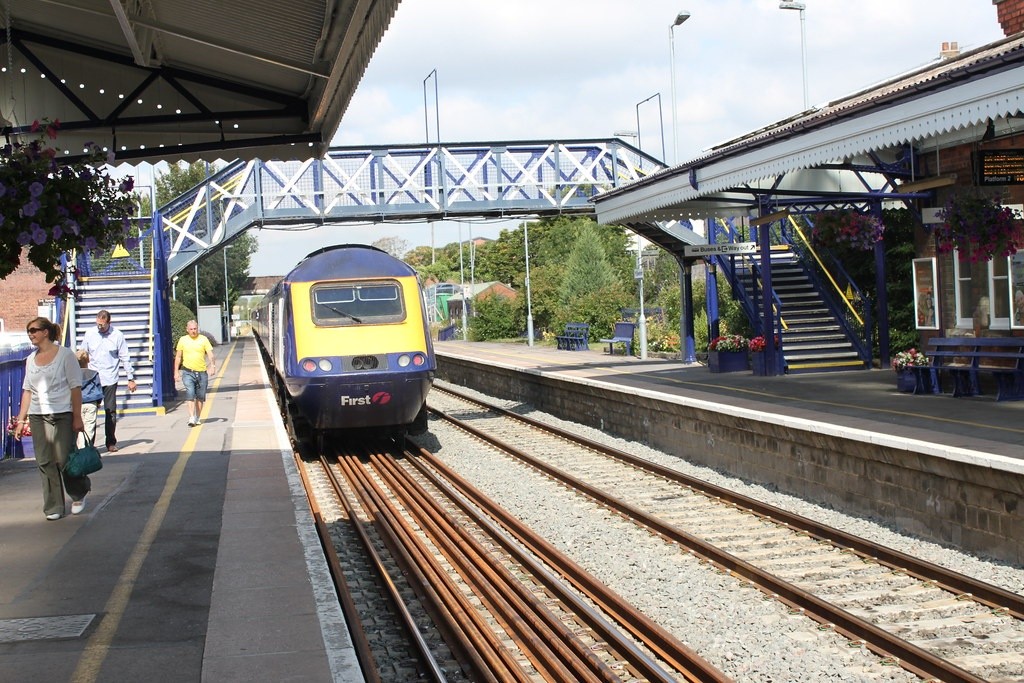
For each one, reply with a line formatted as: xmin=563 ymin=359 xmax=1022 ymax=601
xmin=708 ymin=335 xmax=751 ymax=353
xmin=748 ymin=333 xmax=779 ymax=352
xmin=812 ymin=210 xmax=885 ymax=252
xmin=5 ymin=415 xmax=32 ymax=437
xmin=893 ymin=348 xmax=929 ymax=373
xmin=0 ymin=114 xmax=152 ymax=302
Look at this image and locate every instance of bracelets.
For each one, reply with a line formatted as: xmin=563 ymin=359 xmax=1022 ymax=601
xmin=18 ymin=420 xmax=25 ymax=424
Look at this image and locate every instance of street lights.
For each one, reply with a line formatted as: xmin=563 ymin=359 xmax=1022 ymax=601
xmin=614 ymin=129 xmax=649 ymax=360
xmin=193 ymin=229 xmax=206 ymax=332
xmin=778 ymin=0 xmax=810 ymax=111
xmin=223 ymin=244 xmax=234 ymax=343
xmin=669 ymin=10 xmax=692 ymax=164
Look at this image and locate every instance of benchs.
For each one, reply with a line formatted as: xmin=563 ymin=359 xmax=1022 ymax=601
xmin=905 ymin=337 xmax=1024 ymax=401
xmin=555 ymin=322 xmax=589 ymax=351
xmin=599 ymin=322 xmax=637 ymax=355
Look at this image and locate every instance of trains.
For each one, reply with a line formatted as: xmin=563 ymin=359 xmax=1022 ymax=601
xmin=247 ymin=243 xmax=438 ymax=452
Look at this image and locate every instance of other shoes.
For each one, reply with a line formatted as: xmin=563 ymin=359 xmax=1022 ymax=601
xmin=45 ymin=513 xmax=60 ymax=520
xmin=107 ymin=442 xmax=118 ymax=452
xmin=72 ymin=490 xmax=89 ymax=514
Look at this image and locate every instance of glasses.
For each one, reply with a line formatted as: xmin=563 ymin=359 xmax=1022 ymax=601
xmin=27 ymin=327 xmax=43 ymax=334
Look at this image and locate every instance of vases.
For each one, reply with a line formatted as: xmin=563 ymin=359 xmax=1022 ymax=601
xmin=898 ymin=368 xmax=926 ymax=393
xmin=750 ymin=352 xmax=779 ymax=376
xmin=708 ymin=350 xmax=749 ymax=373
xmin=15 ymin=436 xmax=34 ymax=458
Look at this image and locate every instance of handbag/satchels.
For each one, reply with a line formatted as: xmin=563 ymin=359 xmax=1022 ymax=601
xmin=62 ymin=429 xmax=103 ymax=478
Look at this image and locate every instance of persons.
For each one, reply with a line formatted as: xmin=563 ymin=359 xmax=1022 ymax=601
xmin=174 ymin=320 xmax=215 ymax=428
xmin=77 ymin=309 xmax=137 ymax=452
xmin=15 ymin=316 xmax=89 ymax=519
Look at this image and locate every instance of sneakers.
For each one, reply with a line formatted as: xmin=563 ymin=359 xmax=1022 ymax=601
xmin=187 ymin=415 xmax=202 ymax=426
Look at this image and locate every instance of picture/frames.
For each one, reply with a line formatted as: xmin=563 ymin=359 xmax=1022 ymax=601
xmin=931 ymin=194 xmax=1024 ymax=262
